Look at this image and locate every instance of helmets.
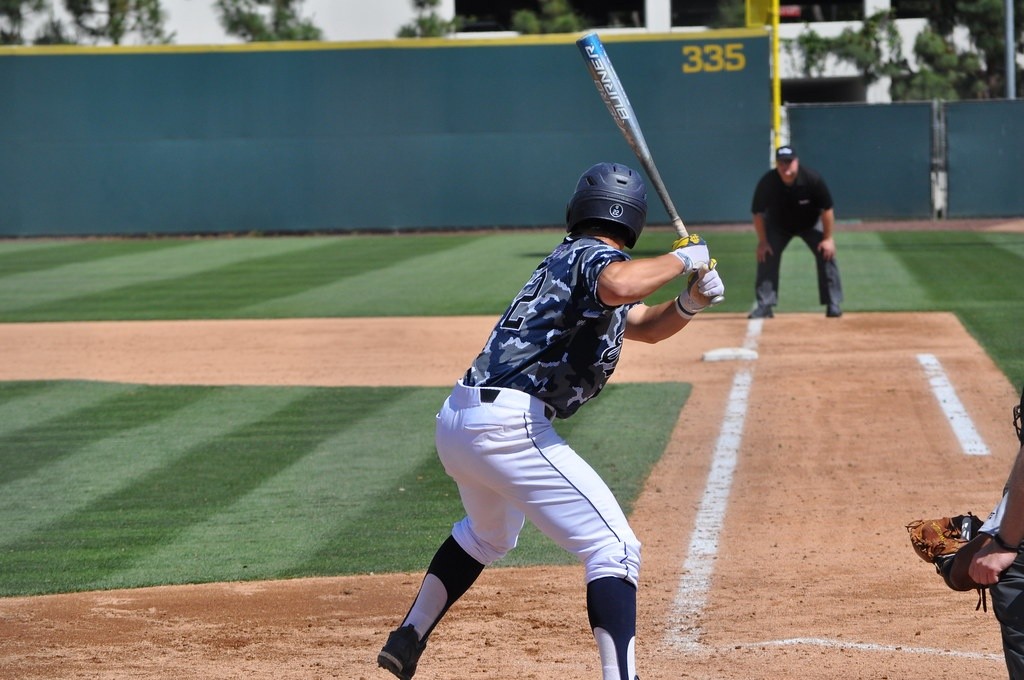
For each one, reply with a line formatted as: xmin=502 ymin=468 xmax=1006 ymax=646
xmin=566 ymin=161 xmax=647 ymax=249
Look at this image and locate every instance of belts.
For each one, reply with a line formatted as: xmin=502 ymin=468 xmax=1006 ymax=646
xmin=480 ymin=389 xmax=553 ymax=421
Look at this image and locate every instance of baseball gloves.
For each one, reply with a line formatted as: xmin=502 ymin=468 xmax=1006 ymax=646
xmin=905 ymin=514 xmax=972 ymax=566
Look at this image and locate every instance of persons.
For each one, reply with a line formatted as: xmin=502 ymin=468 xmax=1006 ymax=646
xmin=748 ymin=145 xmax=843 ymax=318
xmin=907 ymin=382 xmax=1024 ymax=680
xmin=376 ymin=164 xmax=723 ymax=680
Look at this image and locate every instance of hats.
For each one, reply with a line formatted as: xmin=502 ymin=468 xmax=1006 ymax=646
xmin=776 ymin=145 xmax=796 ymax=160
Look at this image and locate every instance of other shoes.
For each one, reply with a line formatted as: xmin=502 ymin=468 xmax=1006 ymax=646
xmin=827 ymin=304 xmax=842 ymax=317
xmin=747 ymin=305 xmax=773 ymax=319
xmin=377 ymin=623 xmax=427 ymax=680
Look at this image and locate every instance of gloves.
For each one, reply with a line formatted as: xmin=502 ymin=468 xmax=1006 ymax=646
xmin=668 ymin=234 xmax=710 ymax=275
xmin=675 ymin=258 xmax=725 ymax=319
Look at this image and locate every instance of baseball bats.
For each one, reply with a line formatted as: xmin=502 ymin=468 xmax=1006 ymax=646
xmin=577 ymin=31 xmax=725 ymax=306
